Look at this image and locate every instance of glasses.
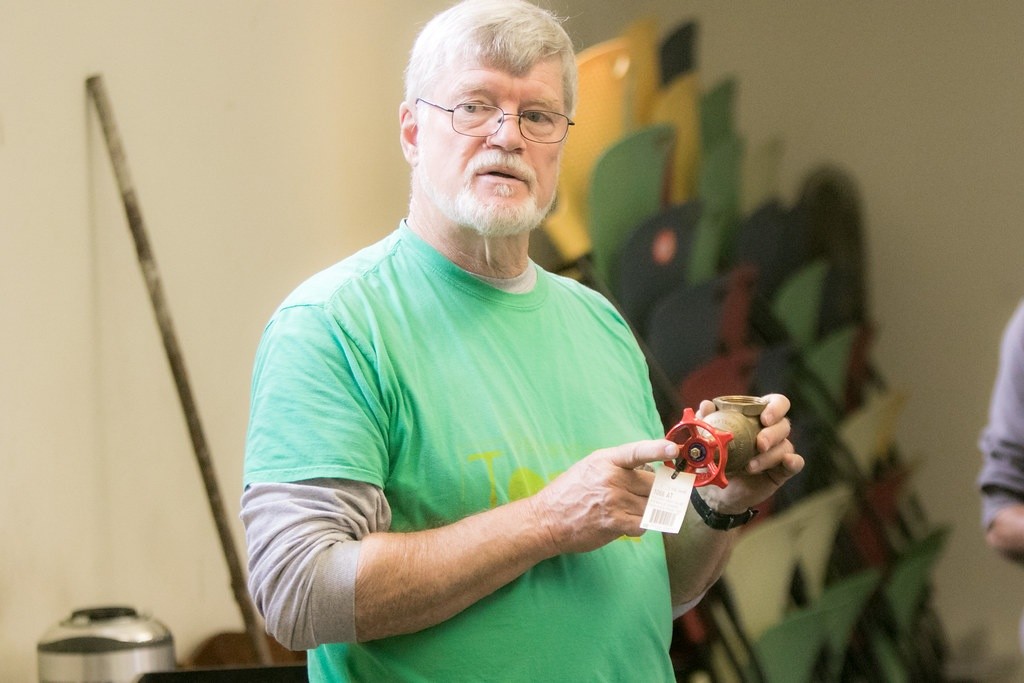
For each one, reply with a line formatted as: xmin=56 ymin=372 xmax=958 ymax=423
xmin=416 ymin=98 xmax=576 ymax=143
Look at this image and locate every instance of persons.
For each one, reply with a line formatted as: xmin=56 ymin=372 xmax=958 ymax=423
xmin=238 ymin=0 xmax=806 ymax=683
xmin=977 ymin=298 xmax=1024 ymax=556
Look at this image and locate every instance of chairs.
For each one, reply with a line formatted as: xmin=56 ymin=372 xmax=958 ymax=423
xmin=535 ymin=15 xmax=954 ymax=682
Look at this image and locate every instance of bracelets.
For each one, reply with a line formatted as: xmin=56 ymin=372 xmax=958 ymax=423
xmin=691 ymin=487 xmax=759 ymax=531
